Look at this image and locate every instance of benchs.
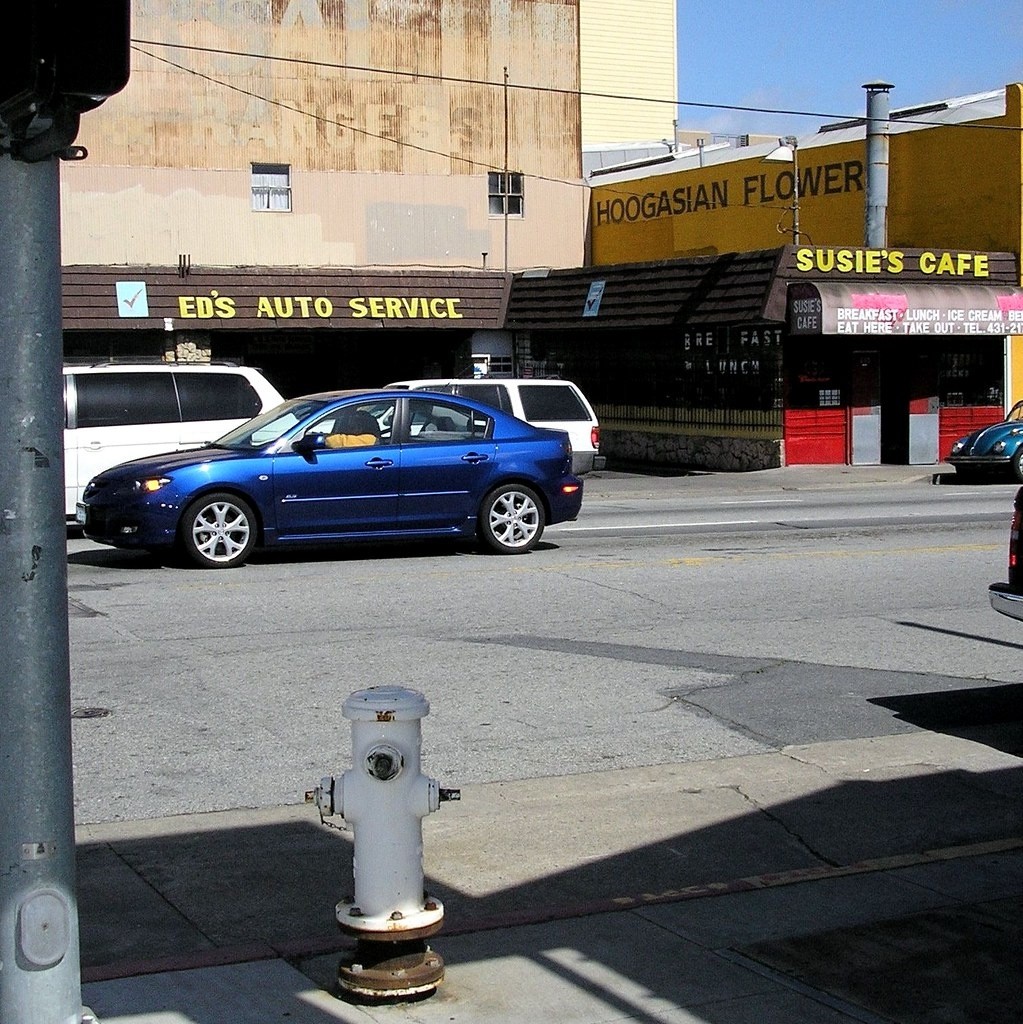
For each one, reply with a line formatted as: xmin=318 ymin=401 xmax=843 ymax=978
xmin=416 ymin=416 xmax=486 ymax=441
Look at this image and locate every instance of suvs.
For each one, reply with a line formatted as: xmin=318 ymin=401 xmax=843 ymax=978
xmin=307 ymin=379 xmax=607 ymax=479
xmin=58 ymin=356 xmax=310 ymax=535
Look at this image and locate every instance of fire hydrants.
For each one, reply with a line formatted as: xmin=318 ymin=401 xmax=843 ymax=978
xmin=304 ymin=683 xmax=464 ymax=1007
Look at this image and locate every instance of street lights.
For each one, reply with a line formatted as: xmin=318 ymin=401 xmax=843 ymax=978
xmin=765 ymin=144 xmax=800 ymax=242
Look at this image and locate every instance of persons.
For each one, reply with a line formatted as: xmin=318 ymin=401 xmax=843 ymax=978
xmin=324 ymin=410 xmax=380 ymax=449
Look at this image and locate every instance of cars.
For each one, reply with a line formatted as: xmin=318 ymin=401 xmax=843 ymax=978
xmin=77 ymin=388 xmax=582 ymax=570
xmin=945 ymin=400 xmax=1022 ymax=482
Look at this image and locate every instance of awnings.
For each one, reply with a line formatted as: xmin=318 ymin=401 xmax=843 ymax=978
xmin=785 ymin=283 xmax=1023 ymax=335
